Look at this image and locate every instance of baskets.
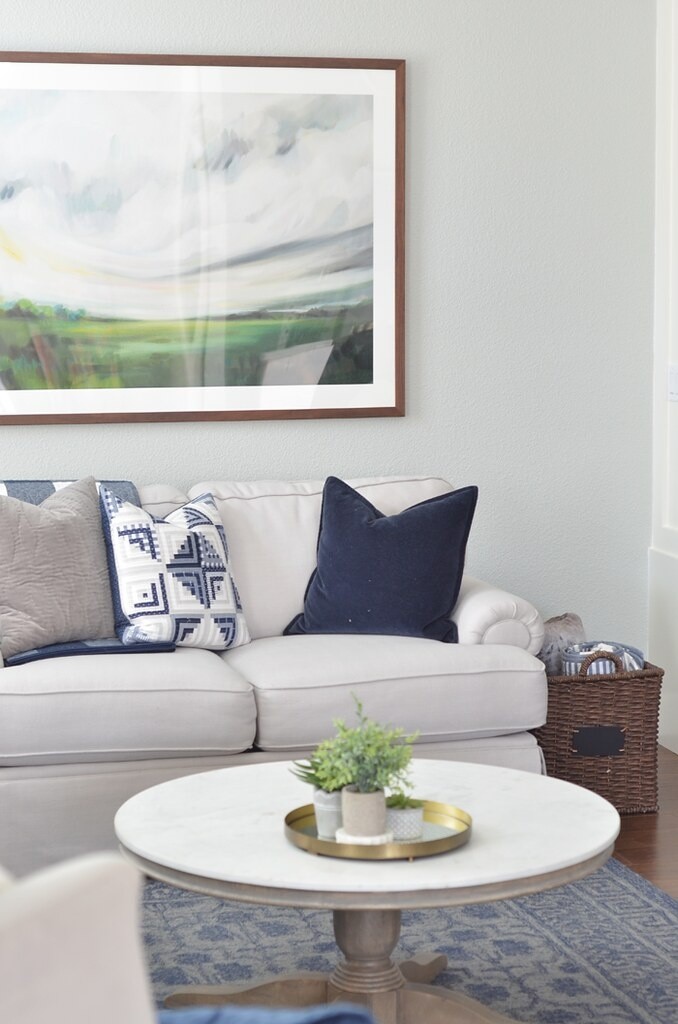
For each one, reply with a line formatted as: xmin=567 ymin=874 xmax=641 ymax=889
xmin=527 ymin=651 xmax=664 ymax=813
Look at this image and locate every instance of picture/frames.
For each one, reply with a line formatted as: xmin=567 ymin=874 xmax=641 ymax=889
xmin=0 ymin=52 xmax=405 ymax=427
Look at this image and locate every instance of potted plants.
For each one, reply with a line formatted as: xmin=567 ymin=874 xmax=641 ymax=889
xmin=289 ymin=690 xmax=425 ymax=845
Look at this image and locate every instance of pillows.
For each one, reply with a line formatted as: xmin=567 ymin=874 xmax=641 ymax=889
xmin=281 ymin=476 xmax=478 ymax=641
xmin=0 ymin=477 xmax=116 ymax=661
xmin=98 ymin=486 xmax=252 ymax=651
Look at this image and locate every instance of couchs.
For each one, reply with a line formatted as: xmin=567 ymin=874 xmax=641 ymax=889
xmin=0 ymin=477 xmax=550 ymax=884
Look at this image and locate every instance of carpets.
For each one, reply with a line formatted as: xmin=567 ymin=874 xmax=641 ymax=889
xmin=141 ymin=856 xmax=678 ymax=1024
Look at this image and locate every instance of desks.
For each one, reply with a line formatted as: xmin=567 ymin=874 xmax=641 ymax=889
xmin=114 ymin=758 xmax=622 ymax=1024
